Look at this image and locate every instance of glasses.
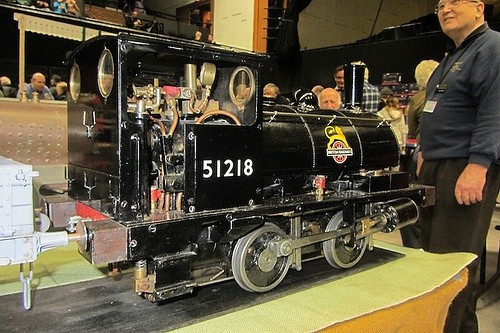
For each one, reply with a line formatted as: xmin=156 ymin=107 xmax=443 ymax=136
xmin=434 ymin=0 xmax=478 ymax=15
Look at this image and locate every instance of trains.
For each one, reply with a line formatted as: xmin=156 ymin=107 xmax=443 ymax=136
xmin=0 ymin=29 xmax=438 ymax=311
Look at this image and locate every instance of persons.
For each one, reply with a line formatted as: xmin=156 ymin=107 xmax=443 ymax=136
xmin=276 ymin=61 xmax=344 ymax=111
xmin=0 ymin=76 xmax=17 ymax=97
xmin=6 ymin=0 xmax=81 ymax=17
xmin=263 ymin=83 xmax=280 ymax=99
xmin=208 ymin=33 xmax=213 ymax=44
xmin=71 ymin=81 xmax=81 ymax=103
xmin=132 ymin=0 xmax=147 ymax=15
xmin=415 ymin=0 xmax=500 ymax=333
xmin=235 ymin=84 xmax=251 ymax=102
xmin=350 ymin=61 xmax=408 ymax=173
xmin=408 ymin=58 xmax=441 ymax=161
xmin=122 ymin=0 xmax=131 ymax=13
xmin=101 ymin=74 xmax=113 ymax=96
xmin=16 ymin=71 xmax=68 ymax=102
xmin=194 ymin=30 xmax=202 ymax=41
